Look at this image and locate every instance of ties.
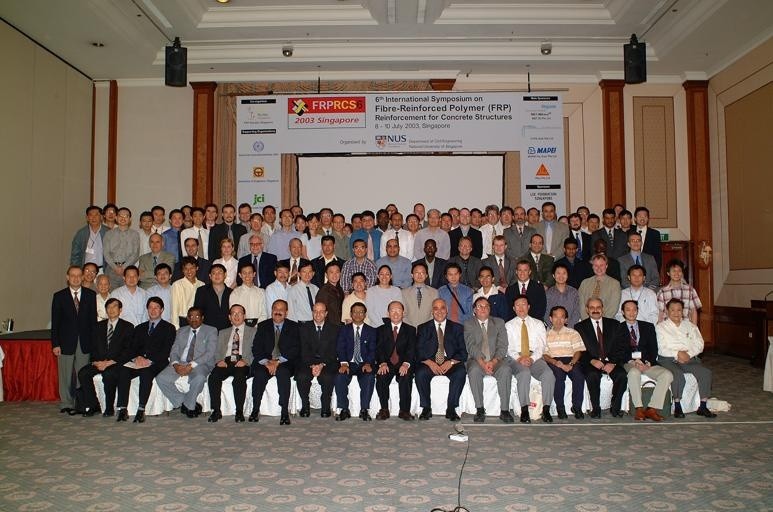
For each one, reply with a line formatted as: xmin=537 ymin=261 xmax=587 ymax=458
xmin=252 ymin=255 xmax=258 ymax=286
xmin=395 ymin=231 xmax=399 ymax=247
xmin=274 ymin=326 xmax=281 ymax=354
xmin=637 ymin=230 xmax=642 ymax=253
xmin=591 ymin=280 xmax=600 ymax=297
xmin=518 ymin=227 xmax=524 ymax=239
xmin=198 ymin=230 xmax=204 ymax=259
xmin=289 ymin=259 xmax=298 ymax=283
xmin=635 ymin=256 xmax=640 ymax=265
xmin=390 ymin=326 xmax=399 ymax=365
xmin=575 ymin=232 xmax=582 ymax=259
xmin=317 ymin=326 xmax=322 ymax=343
xmin=228 ymin=224 xmax=234 ymax=250
xmin=306 ymin=286 xmax=314 ymax=310
xmin=595 ymin=320 xmax=604 ymax=358
xmin=630 ymin=325 xmax=637 ymax=345
xmin=451 ymin=288 xmax=458 ymax=321
xmin=435 ymin=323 xmax=445 ymax=365
xmin=480 ymin=323 xmax=490 ymax=363
xmin=416 ymin=287 xmax=421 ymax=308
xmin=107 ymin=324 xmax=114 ymax=342
xmin=153 ymin=255 xmax=157 ymax=268
xmin=545 ymin=223 xmax=552 ymax=255
xmin=498 ymin=259 xmax=504 ymax=281
xmin=520 ymin=319 xmax=530 ymax=357
xmin=326 ymin=229 xmax=330 ymax=235
xmin=176 ymin=231 xmax=183 ymax=262
xmin=608 ymin=229 xmax=614 ymax=247
xmin=186 ymin=329 xmax=197 ymax=362
xmin=74 ymin=292 xmax=80 ymax=314
xmin=231 ymin=328 xmax=240 ymax=357
xmin=521 ymin=283 xmax=526 ymax=295
xmin=354 ymin=326 xmax=361 ymax=365
xmin=148 ymin=322 xmax=156 ymax=335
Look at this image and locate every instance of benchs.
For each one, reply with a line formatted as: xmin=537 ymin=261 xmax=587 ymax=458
xmin=95 ymin=369 xmax=705 ymax=418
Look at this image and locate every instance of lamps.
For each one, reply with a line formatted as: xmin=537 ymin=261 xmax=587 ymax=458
xmin=282 ymin=47 xmax=294 ymax=56
xmin=541 ymin=43 xmax=552 ymax=55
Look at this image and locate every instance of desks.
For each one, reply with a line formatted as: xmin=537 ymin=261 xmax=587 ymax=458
xmin=1 ymin=329 xmax=59 ymax=402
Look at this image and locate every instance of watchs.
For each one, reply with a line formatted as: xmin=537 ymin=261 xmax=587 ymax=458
xmin=569 ymin=362 xmax=575 ymax=366
xmin=450 ymin=359 xmax=455 ymax=365
xmin=276 ymin=359 xmax=280 ymax=363
xmin=188 ymin=363 xmax=192 ymax=369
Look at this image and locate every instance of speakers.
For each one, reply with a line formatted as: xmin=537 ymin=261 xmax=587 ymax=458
xmin=164 ymin=36 xmax=188 ymax=88
xmin=623 ymin=33 xmax=647 ymax=84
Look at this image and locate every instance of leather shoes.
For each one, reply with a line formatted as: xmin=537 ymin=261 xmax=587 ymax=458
xmin=542 ymin=413 xmax=552 ymax=422
xmin=235 ymin=414 xmax=245 ymax=422
xmin=180 ymin=404 xmax=198 ymax=418
xmin=500 ymin=412 xmax=514 ymax=423
xmin=103 ymin=409 xmax=114 ymax=417
xmin=610 ymin=407 xmax=621 ymax=418
xmin=248 ymin=410 xmax=259 ymax=422
xmin=117 ymin=409 xmax=130 ymax=422
xmin=299 ymin=408 xmax=311 ymax=417
xmin=674 ymin=410 xmax=685 ymax=418
xmin=398 ymin=410 xmax=415 ymax=421
xmin=359 ymin=410 xmax=372 ymax=421
xmin=591 ymin=407 xmax=601 ymax=419
xmin=208 ymin=410 xmax=222 ymax=422
xmin=336 ymin=409 xmax=351 ymax=420
xmin=634 ymin=407 xmax=645 ymax=422
xmin=82 ymin=406 xmax=98 ymax=417
xmin=133 ymin=410 xmax=146 ymax=423
xmin=520 ymin=412 xmax=530 ymax=423
xmin=445 ymin=409 xmax=460 ymax=421
xmin=474 ymin=409 xmax=485 ymax=422
xmin=556 ymin=406 xmax=568 ymax=419
xmin=376 ymin=408 xmax=390 ymax=420
xmin=279 ymin=413 xmax=290 ymax=425
xmin=645 ymin=409 xmax=665 ymax=421
xmin=570 ymin=406 xmax=585 ymax=419
xmin=419 ymin=407 xmax=432 ymax=420
xmin=321 ymin=408 xmax=331 ymax=417
xmin=696 ymin=407 xmax=717 ymax=418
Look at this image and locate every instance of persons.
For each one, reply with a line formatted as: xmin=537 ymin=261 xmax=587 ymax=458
xmin=448 ymin=208 xmax=483 ymax=260
xmin=542 ymin=306 xmax=586 ymax=420
xmin=505 ymin=260 xmax=546 ymax=318
xmin=405 ymin=214 xmax=420 ymax=235
xmin=375 ymin=239 xmax=414 ymax=288
xmin=375 ymin=210 xmax=390 ymax=232
xmin=574 ymin=239 xmax=620 ymax=289
xmin=208 ymin=204 xmax=247 ymax=262
xmin=162 ymin=209 xmax=185 ymax=262
xmin=295 ymin=215 xmax=309 ymax=234
xmin=142 ymin=263 xmax=172 ymax=323
xmin=116 ymin=296 xmax=176 ymax=422
xmin=448 ymin=207 xmax=459 ymax=231
xmin=301 ymin=213 xmax=324 ymax=261
xmin=505 ymin=295 xmax=556 ymax=424
xmin=655 ymin=298 xmax=718 ymax=418
xmin=281 ymin=236 xmax=310 ymax=285
xmin=208 ymin=305 xmax=258 ymax=424
xmin=71 ymin=206 xmax=111 ymax=274
xmin=559 ymin=216 xmax=569 ymax=226
xmin=473 ymin=266 xmax=511 ymax=322
xmin=482 ymin=236 xmax=518 ymax=292
xmin=289 ymin=262 xmax=320 ymax=323
xmin=329 ymin=214 xmax=352 ymax=262
xmin=616 ymin=210 xmax=636 ymax=235
xmin=577 ymin=206 xmax=590 ymax=232
xmin=293 ymin=303 xmax=339 ymax=418
xmin=266 ymin=260 xmax=293 ymax=321
xmin=156 ymin=307 xmax=219 ymax=418
xmin=180 ymin=207 xmax=209 ymax=259
xmin=137 ymin=234 xmax=176 ymax=288
xmin=238 ymin=212 xmax=270 ymax=258
xmin=333 ymin=303 xmax=376 ymax=421
xmin=380 ymin=213 xmax=417 ymax=263
xmin=239 ymin=203 xmax=254 ymax=232
xmin=628 ymin=208 xmax=662 ymax=274
xmin=500 ymin=207 xmax=513 ymax=229
xmin=342 ymin=224 xmax=353 ymax=239
xmin=96 ymin=274 xmax=111 ymax=321
xmin=375 ymin=303 xmax=416 ymax=421
xmin=260 ymin=206 xmax=281 ymax=236
xmin=271 ymin=209 xmax=303 ymax=261
xmin=479 ymin=206 xmax=504 ymax=259
xmin=111 ymin=265 xmax=145 ymax=326
xmin=585 ymin=213 xmax=600 ymax=233
xmin=229 ymin=262 xmax=266 ymax=328
xmin=403 ymin=204 xmax=428 ymax=232
xmin=215 ymin=239 xmax=240 ymax=289
xmin=536 ymin=203 xmax=569 ymax=262
xmin=470 ymin=208 xmax=482 ymax=229
xmin=616 ymin=265 xmax=659 ymax=325
xmin=556 ymin=237 xmax=583 ymax=287
xmin=351 ymin=214 xmax=361 ymax=231
xmin=161 ymin=211 xmax=172 ymax=256
xmin=203 ymin=203 xmax=219 ymax=229
xmin=503 ymin=206 xmax=538 ymax=262
xmin=415 ymin=299 xmax=468 ymax=421
xmin=349 ymin=210 xmax=383 ymax=261
xmin=414 ymin=239 xmax=446 ymax=289
xmin=385 ymin=204 xmax=399 ymax=227
xmin=316 ymin=208 xmax=334 ymax=236
xmin=401 ymin=260 xmax=438 ymax=326
xmin=614 ymin=205 xmax=624 ymax=229
xmin=194 ymin=264 xmax=234 ymax=330
xmin=448 ymin=236 xmax=481 ymax=293
xmin=527 ymin=207 xmax=540 ymax=226
xmin=520 ymin=234 xmax=555 ymax=290
xmin=574 ymin=296 xmax=627 ymax=418
xmin=341 ymin=272 xmax=367 ymax=325
xmin=620 ymin=300 xmax=673 ymax=421
xmin=438 ymin=262 xmax=475 ymax=324
xmin=78 ymin=298 xmax=134 ymax=417
xmin=82 ymin=263 xmax=99 ymax=294
xmin=289 ymin=206 xmax=303 ymax=220
xmin=568 ymin=212 xmax=591 ymax=263
xmin=590 ymin=209 xmax=629 ymax=260
xmin=172 ymin=239 xmax=212 ymax=283
xmin=137 ymin=210 xmax=156 ymax=254
xmin=102 ymin=207 xmax=139 ymax=274
xmin=657 ymin=259 xmax=702 ymax=325
xmin=151 ymin=206 xmax=170 ymax=234
xmin=249 ymin=301 xmax=301 ymax=425
xmin=440 ymin=213 xmax=452 ymax=234
xmin=181 ymin=205 xmax=193 ymax=228
xmin=365 ymin=265 xmax=403 ymax=327
xmin=578 ymin=254 xmax=621 ymax=321
xmin=51 ymin=266 xmax=97 ymax=415
xmin=414 ymin=209 xmax=451 ymax=261
xmin=102 ymin=204 xmax=119 ymax=228
xmin=315 ymin=261 xmax=345 ymax=324
xmin=236 ymin=237 xmax=277 ymax=289
xmin=339 ymin=239 xmax=377 ymax=296
xmin=481 ymin=213 xmax=489 ymax=227
xmin=544 ymin=263 xmax=579 ymax=330
xmin=170 ymin=256 xmax=205 ymax=330
xmin=464 ymin=297 xmax=514 ymax=423
xmin=310 ymin=235 xmax=345 ymax=290
xmin=618 ymin=231 xmax=660 ymax=293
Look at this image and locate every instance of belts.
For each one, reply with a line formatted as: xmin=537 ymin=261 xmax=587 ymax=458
xmin=114 ymin=261 xmax=125 ymax=266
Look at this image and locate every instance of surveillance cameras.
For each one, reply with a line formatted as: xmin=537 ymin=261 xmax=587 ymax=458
xmin=282 ymin=46 xmax=293 ymax=57
xmin=540 ymin=44 xmax=552 ymax=55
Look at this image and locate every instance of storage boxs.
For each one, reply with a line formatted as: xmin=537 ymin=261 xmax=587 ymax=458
xmin=750 ymin=300 xmax=773 ymax=369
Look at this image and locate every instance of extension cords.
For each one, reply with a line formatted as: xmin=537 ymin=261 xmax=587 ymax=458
xmin=448 ymin=433 xmax=470 ymax=442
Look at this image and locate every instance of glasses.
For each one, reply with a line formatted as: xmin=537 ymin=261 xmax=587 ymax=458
xmin=230 ymin=312 xmax=243 ymax=316
xmin=118 ymin=213 xmax=129 ymax=218
xmin=106 ymin=210 xmax=116 ymax=214
xmin=188 ymin=315 xmax=201 ymax=320
xmin=85 ymin=268 xmax=97 ymax=274
